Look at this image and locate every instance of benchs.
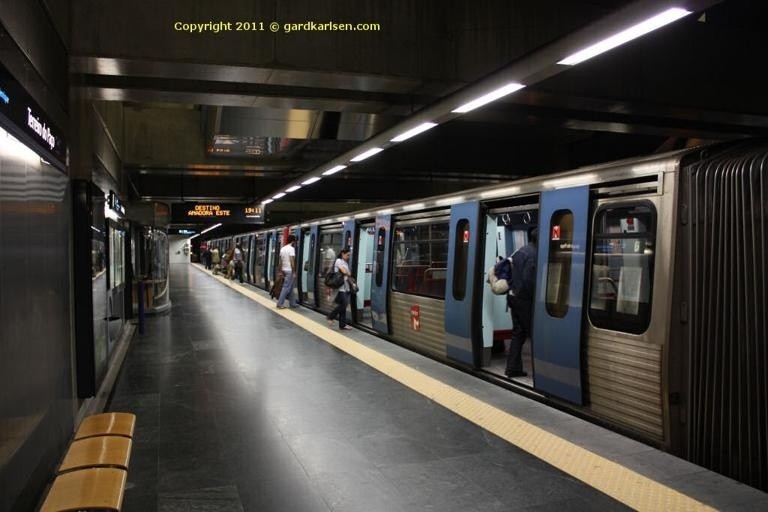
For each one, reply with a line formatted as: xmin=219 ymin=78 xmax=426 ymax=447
xmin=38 ymin=410 xmax=139 ymax=511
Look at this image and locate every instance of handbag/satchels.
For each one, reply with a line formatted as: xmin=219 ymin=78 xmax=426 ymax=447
xmin=324 ymin=272 xmax=344 ymax=288
xmin=349 ymin=281 xmax=359 ymax=294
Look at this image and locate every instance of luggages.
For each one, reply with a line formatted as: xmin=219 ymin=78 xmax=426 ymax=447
xmin=270 ymin=272 xmax=285 ymax=300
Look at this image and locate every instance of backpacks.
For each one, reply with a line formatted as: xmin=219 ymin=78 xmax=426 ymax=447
xmin=486 ymin=255 xmax=514 ymax=295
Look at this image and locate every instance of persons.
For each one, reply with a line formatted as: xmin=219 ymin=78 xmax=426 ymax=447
xmin=226 ymin=248 xmax=235 ymax=279
xmin=221 ymin=247 xmax=229 ymax=278
xmin=495 ymin=226 xmax=537 ymax=379
xmin=275 ymin=234 xmax=301 ymax=309
xmin=324 ymin=247 xmax=355 ymax=330
xmin=229 ymin=242 xmax=247 ymax=285
xmin=208 ymin=245 xmax=220 ymax=275
xmin=199 ymin=247 xmax=212 ymax=270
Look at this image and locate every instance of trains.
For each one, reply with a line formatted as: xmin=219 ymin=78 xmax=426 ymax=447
xmin=199 ymin=131 xmax=768 ymax=497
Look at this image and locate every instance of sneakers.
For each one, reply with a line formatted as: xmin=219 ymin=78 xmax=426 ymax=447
xmin=339 ymin=324 xmax=352 ymax=330
xmin=225 ymin=276 xmax=235 ymax=281
xmin=205 ymin=267 xmax=211 ymax=270
xmin=504 ymin=369 xmax=527 ymax=378
xmin=326 ymin=317 xmax=335 ymax=329
xmin=276 ymin=304 xmax=299 ymax=309
xmin=212 ymin=272 xmax=220 ymax=275
xmin=239 ymin=283 xmax=247 ymax=285
